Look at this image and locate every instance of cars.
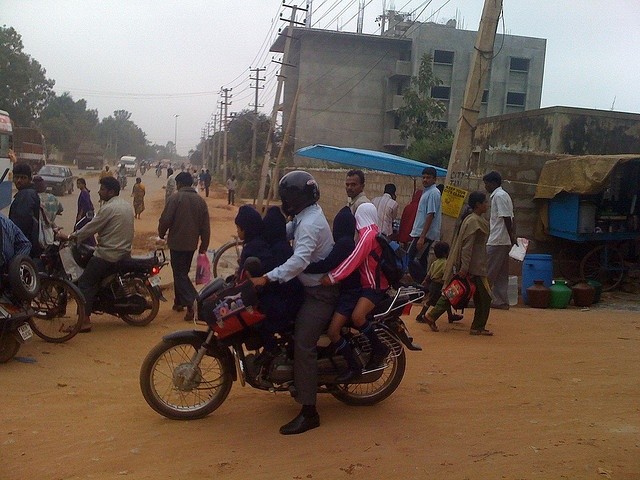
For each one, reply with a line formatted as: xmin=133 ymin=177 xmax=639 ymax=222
xmin=34 ymin=165 xmax=75 ymax=194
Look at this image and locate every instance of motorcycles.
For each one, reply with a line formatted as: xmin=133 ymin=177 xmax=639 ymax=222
xmin=39 ymin=211 xmax=169 ymax=327
xmin=141 ymin=164 xmax=147 ymax=175
xmin=157 ymin=168 xmax=162 ymax=177
xmin=0 ymin=252 xmax=40 ymax=364
xmin=139 ymin=257 xmax=426 ymax=420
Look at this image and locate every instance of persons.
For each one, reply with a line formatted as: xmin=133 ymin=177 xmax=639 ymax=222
xmin=415 ymin=241 xmax=464 ymax=324
xmin=198 ymin=170 xmax=205 ymax=188
xmin=157 ymin=171 xmax=211 ymax=321
xmin=161 ymin=168 xmax=175 ymax=207
xmin=406 ymin=168 xmax=442 ymax=303
xmin=232 ymin=205 xmax=290 ymax=364
xmin=73 ymin=178 xmax=97 ymax=246
xmin=234 ymin=171 xmax=338 ymax=434
xmin=32 ymin=175 xmax=63 ymax=250
xmin=130 ymin=177 xmax=145 ymax=220
xmin=318 ymin=202 xmax=392 ymax=374
xmin=370 ymin=183 xmax=400 ymax=238
xmin=302 ymin=206 xmax=362 ymax=382
xmin=261 ymin=206 xmax=306 ymax=361
xmin=55 ymin=177 xmax=134 ymax=333
xmin=345 ymin=169 xmax=378 ymax=239
xmin=202 ymin=170 xmax=211 ymax=198
xmin=482 ymin=170 xmax=519 ymax=310
xmin=8 ymin=163 xmax=40 ymax=257
xmin=140 ymin=159 xmax=191 ymax=170
xmin=115 ymin=162 xmax=128 ymax=176
xmin=191 ymin=169 xmax=199 ymax=187
xmin=0 ymin=211 xmax=33 ymax=275
xmin=397 ymin=189 xmax=423 ymax=243
xmin=226 ymin=175 xmax=239 ymax=206
xmin=423 ymin=191 xmax=494 ymax=337
xmin=100 ymin=165 xmax=113 ymax=178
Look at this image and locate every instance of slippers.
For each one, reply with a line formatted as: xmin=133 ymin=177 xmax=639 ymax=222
xmin=470 ymin=327 xmax=495 ymax=335
xmin=421 ymin=314 xmax=440 ymax=332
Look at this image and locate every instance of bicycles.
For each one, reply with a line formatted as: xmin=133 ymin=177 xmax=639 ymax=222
xmin=27 ymin=261 xmax=86 ymax=344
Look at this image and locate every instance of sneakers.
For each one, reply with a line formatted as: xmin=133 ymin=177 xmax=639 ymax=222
xmin=416 ymin=314 xmax=424 ymax=322
xmin=448 ymin=313 xmax=463 ymax=323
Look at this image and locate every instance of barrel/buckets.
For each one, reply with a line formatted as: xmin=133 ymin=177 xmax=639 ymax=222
xmin=577 ymin=206 xmax=596 ymax=233
xmin=521 ymin=253 xmax=555 ymax=304
xmin=507 ymin=272 xmax=519 ymax=306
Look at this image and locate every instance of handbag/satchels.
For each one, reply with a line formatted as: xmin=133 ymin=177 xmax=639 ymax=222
xmin=38 ymin=204 xmax=54 ymax=249
xmin=196 ymin=252 xmax=211 ymax=285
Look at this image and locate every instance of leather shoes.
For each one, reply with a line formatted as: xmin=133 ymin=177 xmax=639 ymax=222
xmin=335 ymin=351 xmax=364 ymax=384
xmin=280 ymin=414 xmax=320 ymax=434
xmin=365 ymin=345 xmax=390 ymax=373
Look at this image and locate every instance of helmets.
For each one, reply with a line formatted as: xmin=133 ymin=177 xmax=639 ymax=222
xmin=279 ymin=170 xmax=320 ymax=216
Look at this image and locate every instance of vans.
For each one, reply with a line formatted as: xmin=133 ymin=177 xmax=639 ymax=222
xmin=118 ymin=156 xmax=138 ymax=177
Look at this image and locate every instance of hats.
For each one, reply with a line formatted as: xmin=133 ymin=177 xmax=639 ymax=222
xmin=12 ymin=164 xmax=31 ymax=176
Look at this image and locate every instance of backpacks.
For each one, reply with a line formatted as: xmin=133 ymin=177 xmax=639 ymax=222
xmin=370 ymin=232 xmax=409 ymax=291
xmin=444 ymin=271 xmax=476 ymax=314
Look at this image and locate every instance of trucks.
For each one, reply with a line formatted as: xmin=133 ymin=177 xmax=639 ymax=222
xmin=79 ymin=141 xmax=102 ymax=169
xmin=14 ymin=126 xmax=46 ymax=169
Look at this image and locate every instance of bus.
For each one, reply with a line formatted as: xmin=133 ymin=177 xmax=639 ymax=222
xmin=0 ymin=110 xmax=12 ymax=208
